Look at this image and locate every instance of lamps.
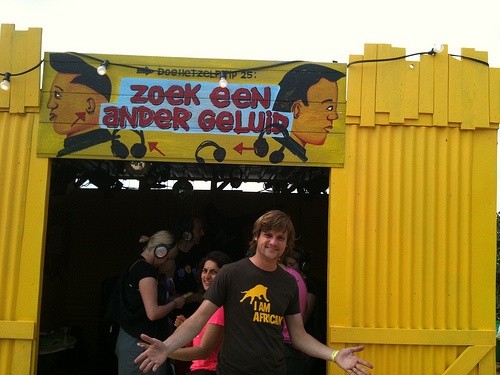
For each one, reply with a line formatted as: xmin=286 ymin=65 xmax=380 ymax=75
xmin=172 ymin=179 xmax=193 ymax=201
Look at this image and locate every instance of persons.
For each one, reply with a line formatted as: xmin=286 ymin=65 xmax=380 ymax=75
xmin=247 ymin=239 xmax=316 ymax=375
xmin=137 ymin=251 xmax=225 ymax=375
xmin=135 ymin=210 xmax=374 ymax=375
xmin=114 ymin=214 xmax=204 ymax=375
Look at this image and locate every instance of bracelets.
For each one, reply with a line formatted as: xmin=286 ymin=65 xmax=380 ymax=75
xmin=331 ymin=349 xmax=341 ymax=362
xmin=173 ymin=300 xmax=177 ymax=309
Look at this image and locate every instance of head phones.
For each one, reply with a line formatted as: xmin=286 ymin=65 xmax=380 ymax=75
xmin=297 ymin=248 xmax=312 ymax=273
xmin=182 ymin=217 xmax=194 ymax=241
xmin=153 ymin=241 xmax=177 ymax=258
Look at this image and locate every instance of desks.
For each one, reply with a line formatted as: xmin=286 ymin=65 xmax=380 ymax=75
xmin=39 ymin=333 xmax=76 ymax=355
